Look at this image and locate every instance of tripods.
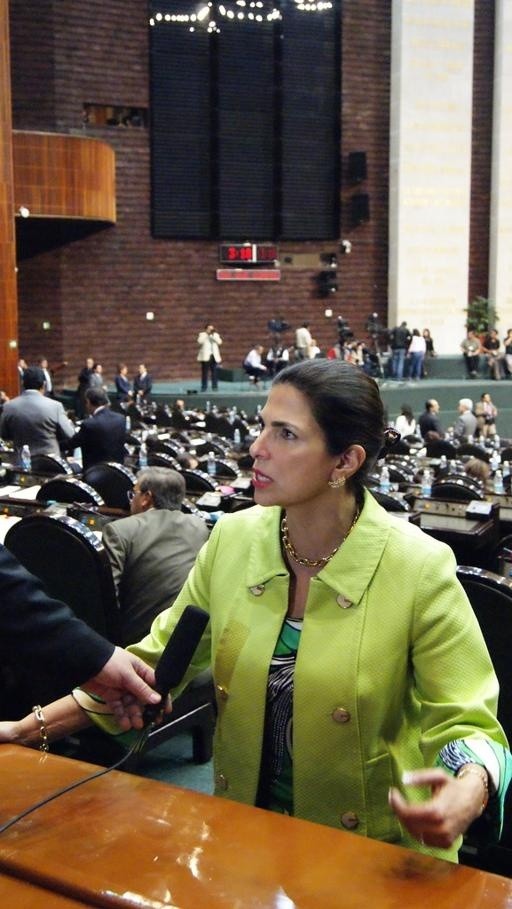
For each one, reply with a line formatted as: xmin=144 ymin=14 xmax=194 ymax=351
xmin=369 ymin=340 xmax=387 ymax=379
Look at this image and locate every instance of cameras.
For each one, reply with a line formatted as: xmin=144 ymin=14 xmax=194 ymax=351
xmin=211 ymin=329 xmax=215 ymax=335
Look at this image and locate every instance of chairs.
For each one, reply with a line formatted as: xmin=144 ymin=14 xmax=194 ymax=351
xmin=1 ymin=402 xmax=512 ymax=748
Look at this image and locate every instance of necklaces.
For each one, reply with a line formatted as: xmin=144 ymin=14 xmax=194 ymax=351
xmin=280 ymin=501 xmax=363 ymax=568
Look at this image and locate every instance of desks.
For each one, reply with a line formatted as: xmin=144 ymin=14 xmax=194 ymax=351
xmin=1 ymin=740 xmax=511 ymax=908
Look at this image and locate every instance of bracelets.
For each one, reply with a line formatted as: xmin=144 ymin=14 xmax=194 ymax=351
xmin=32 ymin=704 xmax=49 ymax=752
xmin=458 ymin=767 xmax=488 ymax=812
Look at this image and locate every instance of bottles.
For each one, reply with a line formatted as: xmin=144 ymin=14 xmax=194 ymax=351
xmin=138 ymin=442 xmax=148 ymax=469
xmin=125 ymin=392 xmax=264 ymax=448
xmin=412 ymin=424 xmax=512 ymax=495
xmin=421 ymin=469 xmax=433 ymax=498
xmin=205 ymin=449 xmax=216 ymax=477
xmin=19 ymin=444 xmax=33 ymax=476
xmin=379 ymin=466 xmax=392 ymax=494
xmin=204 ymin=510 xmax=224 ymax=521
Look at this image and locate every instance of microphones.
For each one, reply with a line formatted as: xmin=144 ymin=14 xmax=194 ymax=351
xmin=143 ymin=604 xmax=210 ymax=721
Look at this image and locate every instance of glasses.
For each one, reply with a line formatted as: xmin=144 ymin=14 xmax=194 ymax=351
xmin=127 ymin=490 xmax=145 ymax=501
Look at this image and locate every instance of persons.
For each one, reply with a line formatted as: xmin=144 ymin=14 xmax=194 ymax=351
xmin=337 ymin=323 xmax=375 ymax=373
xmin=146 ymin=398 xmax=255 ymax=468
xmin=459 ymin=329 xmax=512 ymax=379
xmin=0 ymin=357 xmax=512 ymax=868
xmin=0 ymin=540 xmax=173 ymax=732
xmin=244 ymin=322 xmax=322 ymax=382
xmin=101 ymin=465 xmax=213 ymax=689
xmin=2 ymin=359 xmax=151 ymax=471
xmin=197 ymin=323 xmax=223 ymax=390
xmin=394 ymin=394 xmax=512 ymax=481
xmin=377 ymin=322 xmax=434 ymax=381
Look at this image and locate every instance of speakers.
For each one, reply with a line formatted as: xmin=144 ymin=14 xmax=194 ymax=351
xmin=349 ymin=152 xmax=368 ymax=184
xmin=352 ymin=193 xmax=370 ymax=223
